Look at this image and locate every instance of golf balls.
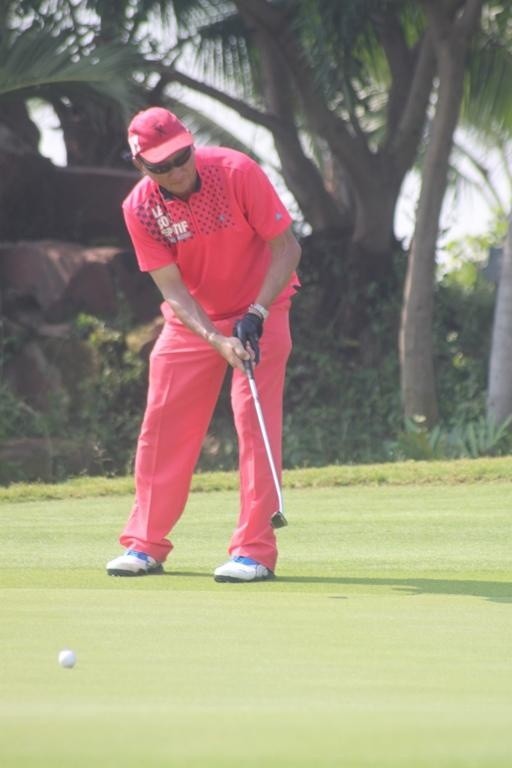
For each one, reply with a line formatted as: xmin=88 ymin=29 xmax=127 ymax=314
xmin=59 ymin=651 xmax=76 ymax=668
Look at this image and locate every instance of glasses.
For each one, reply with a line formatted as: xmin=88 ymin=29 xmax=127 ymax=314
xmin=135 ymin=149 xmax=193 ymax=174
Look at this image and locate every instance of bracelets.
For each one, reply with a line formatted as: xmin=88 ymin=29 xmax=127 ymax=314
xmin=250 ymin=302 xmax=269 ymax=321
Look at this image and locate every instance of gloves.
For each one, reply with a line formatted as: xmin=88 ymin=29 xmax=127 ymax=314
xmin=233 ymin=309 xmax=265 ymax=372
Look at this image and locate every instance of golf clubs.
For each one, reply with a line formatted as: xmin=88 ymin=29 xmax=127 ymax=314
xmin=233 ymin=361 xmax=288 ymax=528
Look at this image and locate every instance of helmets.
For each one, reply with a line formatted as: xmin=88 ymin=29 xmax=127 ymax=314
xmin=125 ymin=106 xmax=194 ymax=167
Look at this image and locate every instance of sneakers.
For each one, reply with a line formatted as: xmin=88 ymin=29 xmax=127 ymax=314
xmin=103 ymin=547 xmax=164 ymax=576
xmin=212 ymin=555 xmax=275 ymax=583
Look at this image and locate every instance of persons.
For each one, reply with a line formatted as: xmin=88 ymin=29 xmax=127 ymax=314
xmin=106 ymin=106 xmax=306 ymax=584
